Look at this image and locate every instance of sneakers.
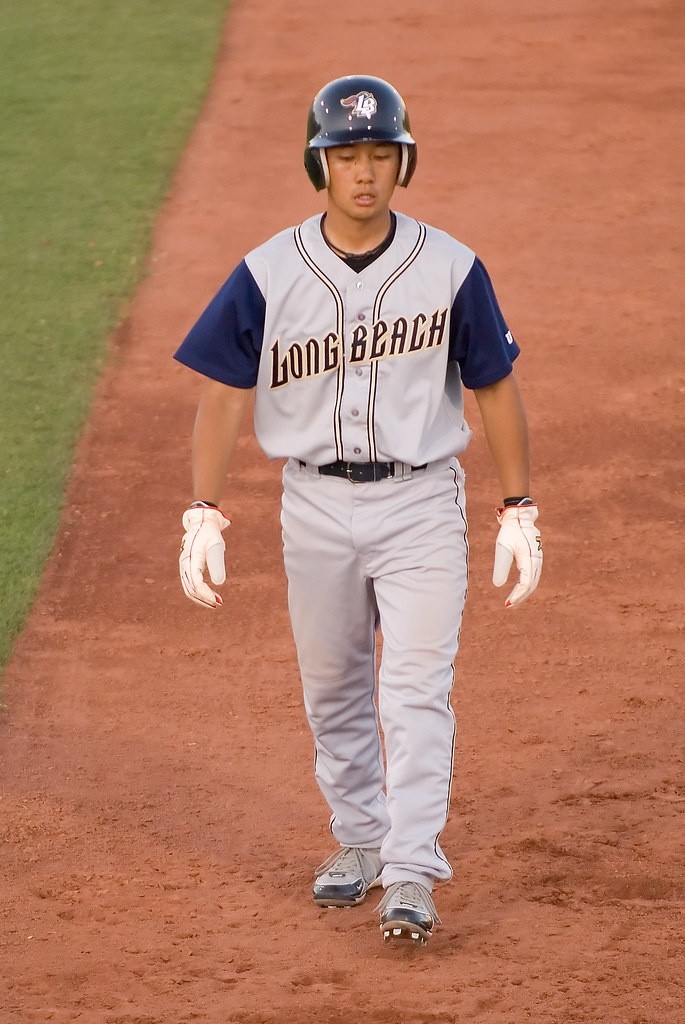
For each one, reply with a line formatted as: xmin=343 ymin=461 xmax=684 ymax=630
xmin=312 ymin=848 xmax=385 ymax=909
xmin=372 ymin=881 xmax=443 ymax=947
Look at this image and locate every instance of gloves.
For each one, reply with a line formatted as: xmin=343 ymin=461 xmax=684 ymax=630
xmin=492 ymin=497 xmax=544 ymax=609
xmin=178 ymin=501 xmax=234 ymax=609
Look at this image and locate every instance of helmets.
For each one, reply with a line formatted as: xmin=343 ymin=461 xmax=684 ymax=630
xmin=303 ymin=75 xmax=418 ymax=192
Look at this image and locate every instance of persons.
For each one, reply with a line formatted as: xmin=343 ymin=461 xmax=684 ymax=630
xmin=172 ymin=75 xmax=543 ymax=944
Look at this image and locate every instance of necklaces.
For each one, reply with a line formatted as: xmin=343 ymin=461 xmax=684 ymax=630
xmin=320 ymin=212 xmax=393 ymax=260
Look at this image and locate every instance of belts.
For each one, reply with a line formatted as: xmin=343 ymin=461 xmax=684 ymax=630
xmin=299 ymin=460 xmax=428 ymax=483
